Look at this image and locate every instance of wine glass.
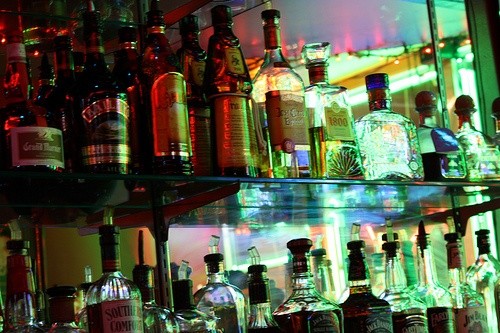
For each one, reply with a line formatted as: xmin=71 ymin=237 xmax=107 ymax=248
xmin=377 ymin=216 xmax=429 ymax=333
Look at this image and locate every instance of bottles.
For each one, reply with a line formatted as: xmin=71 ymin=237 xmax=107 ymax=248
xmin=337 ymin=222 xmax=392 ymax=333
xmin=444 ymin=216 xmax=486 ymax=333
xmin=244 ymin=246 xmax=279 ymax=333
xmin=0 ymin=0 xmax=194 ymax=176
xmin=177 ymin=14 xmax=216 ymax=177
xmin=271 ymin=238 xmax=344 ymax=333
xmin=301 ymin=41 xmax=365 ymax=179
xmin=0 ymin=204 xmax=244 ymax=333
xmin=414 ymin=220 xmax=456 ymax=333
xmin=203 ymin=5 xmax=258 ymax=178
xmin=465 ymin=229 xmax=500 ymax=333
xmin=453 ymin=95 xmax=500 ymax=182
xmin=415 ymin=90 xmax=467 ymax=181
xmin=251 ymin=9 xmax=313 ymax=179
xmin=355 ymin=73 xmax=425 ymax=181
